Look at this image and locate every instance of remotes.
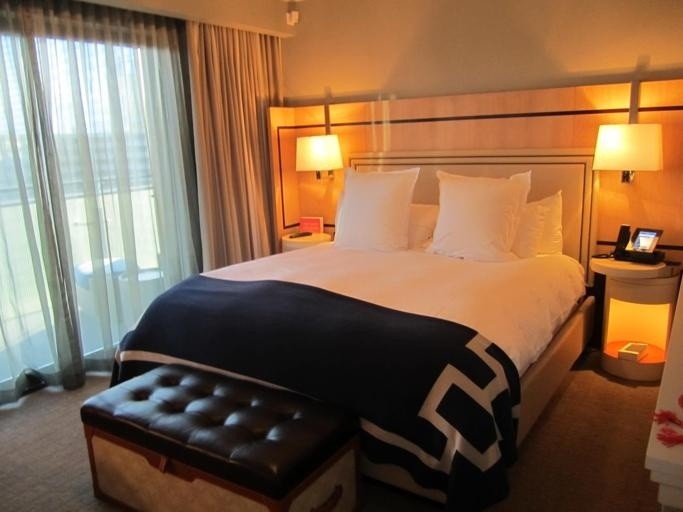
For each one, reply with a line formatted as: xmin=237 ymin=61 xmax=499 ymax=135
xmin=290 ymin=232 xmax=313 ymax=239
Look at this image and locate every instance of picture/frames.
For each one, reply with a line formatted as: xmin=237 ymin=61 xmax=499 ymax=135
xmin=625 ymin=228 xmax=663 ymax=254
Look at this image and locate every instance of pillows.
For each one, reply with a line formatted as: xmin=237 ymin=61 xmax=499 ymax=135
xmin=512 ymin=189 xmax=563 ymax=260
xmin=427 ymin=170 xmax=532 ymax=261
xmin=333 ymin=166 xmax=421 ymax=250
xmin=335 ymin=189 xmax=440 ymax=249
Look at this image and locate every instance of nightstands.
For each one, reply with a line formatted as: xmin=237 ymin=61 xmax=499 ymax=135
xmin=281 ymin=233 xmax=331 ymax=252
xmin=590 ymin=257 xmax=681 ymax=385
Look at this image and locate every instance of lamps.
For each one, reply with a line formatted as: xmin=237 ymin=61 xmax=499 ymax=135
xmin=591 ymin=123 xmax=664 ymax=183
xmin=295 ymin=133 xmax=343 ymax=182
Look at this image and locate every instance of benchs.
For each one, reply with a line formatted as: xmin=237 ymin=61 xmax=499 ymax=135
xmin=80 ymin=362 xmax=364 ymax=512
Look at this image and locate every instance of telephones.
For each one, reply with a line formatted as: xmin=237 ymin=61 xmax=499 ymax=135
xmin=613 ymin=225 xmax=665 ymax=264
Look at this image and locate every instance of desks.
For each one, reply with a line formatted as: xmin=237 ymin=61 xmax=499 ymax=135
xmin=644 ymin=287 xmax=682 ymax=512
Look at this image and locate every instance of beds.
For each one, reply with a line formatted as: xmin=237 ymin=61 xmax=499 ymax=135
xmin=111 ymin=150 xmax=601 ymax=512
